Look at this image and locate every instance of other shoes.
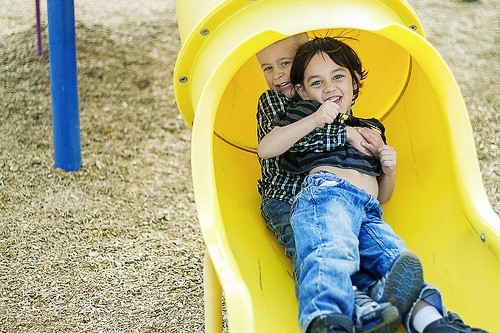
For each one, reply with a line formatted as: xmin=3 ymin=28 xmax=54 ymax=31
xmin=422 ymin=312 xmax=488 ymax=333
xmin=309 ymin=314 xmax=354 ymax=333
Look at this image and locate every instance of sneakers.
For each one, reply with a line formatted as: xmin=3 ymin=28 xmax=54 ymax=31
xmin=367 ymin=251 xmax=423 ymax=317
xmin=352 ymin=290 xmax=399 ymax=333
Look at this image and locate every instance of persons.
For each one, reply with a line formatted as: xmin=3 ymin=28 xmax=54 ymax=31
xmin=256 ymin=32 xmax=423 ymax=333
xmin=257 ymin=36 xmax=488 ymax=333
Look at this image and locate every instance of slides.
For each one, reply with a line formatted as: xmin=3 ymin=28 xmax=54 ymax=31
xmin=164 ymin=0 xmax=500 ymax=333
